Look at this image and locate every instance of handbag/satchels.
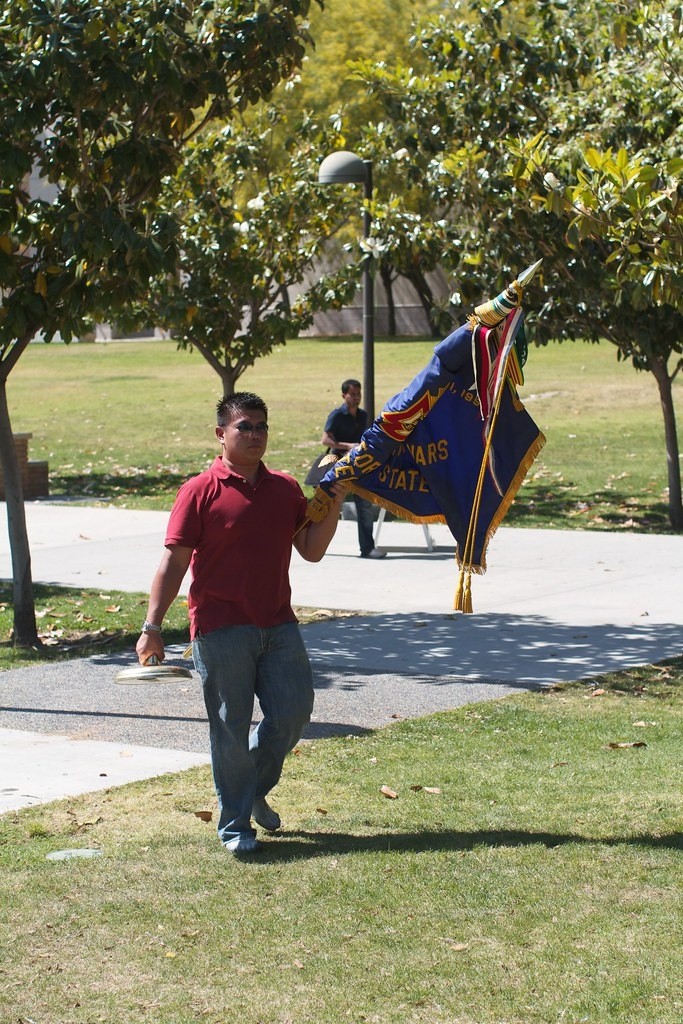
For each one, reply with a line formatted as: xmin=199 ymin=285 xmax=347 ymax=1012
xmin=304 ymin=453 xmax=343 ymax=490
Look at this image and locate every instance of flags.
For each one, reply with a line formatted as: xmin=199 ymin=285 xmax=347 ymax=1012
xmin=305 ymin=281 xmax=547 ymax=614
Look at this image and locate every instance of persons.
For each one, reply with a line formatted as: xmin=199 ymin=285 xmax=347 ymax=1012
xmin=322 ymin=380 xmax=387 ymax=558
xmin=135 ymin=392 xmax=353 ymax=852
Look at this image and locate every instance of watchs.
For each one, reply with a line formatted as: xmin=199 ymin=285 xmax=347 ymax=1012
xmin=141 ymin=621 xmax=162 ymax=632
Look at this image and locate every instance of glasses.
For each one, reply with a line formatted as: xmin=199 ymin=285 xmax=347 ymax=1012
xmin=220 ymin=422 xmax=269 ymax=435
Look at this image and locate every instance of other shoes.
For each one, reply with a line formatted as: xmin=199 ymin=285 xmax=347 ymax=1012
xmin=225 ymin=835 xmax=259 ymax=853
xmin=368 ymin=549 xmax=386 ymax=558
xmin=250 ymin=798 xmax=281 ymax=830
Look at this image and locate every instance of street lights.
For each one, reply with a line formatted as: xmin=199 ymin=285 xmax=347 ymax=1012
xmin=316 ymin=148 xmax=378 ymax=428
